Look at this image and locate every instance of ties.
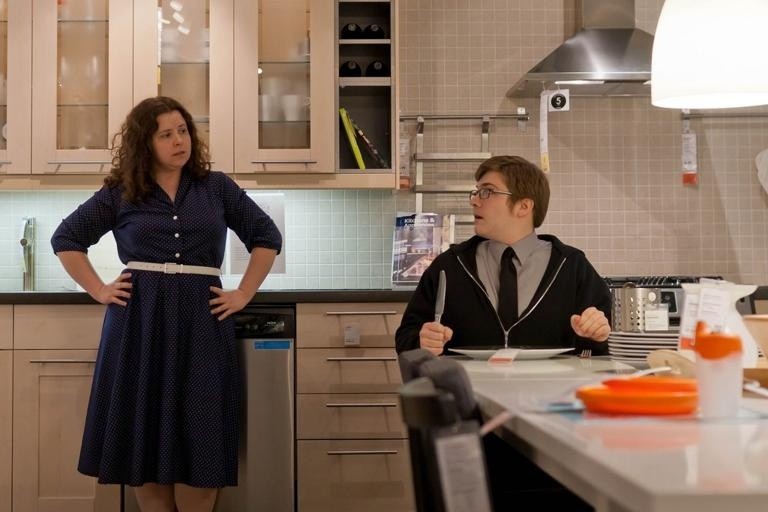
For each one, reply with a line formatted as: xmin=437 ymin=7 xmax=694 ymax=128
xmin=497 ymin=248 xmax=518 ymax=328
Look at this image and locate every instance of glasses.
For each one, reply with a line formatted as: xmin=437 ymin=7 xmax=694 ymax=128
xmin=468 ymin=188 xmax=511 ymax=200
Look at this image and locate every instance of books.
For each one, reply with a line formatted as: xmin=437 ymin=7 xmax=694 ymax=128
xmin=389 ymin=210 xmax=443 ymax=284
xmin=350 ymin=120 xmax=390 ymax=168
xmin=338 ymin=108 xmax=366 ymax=169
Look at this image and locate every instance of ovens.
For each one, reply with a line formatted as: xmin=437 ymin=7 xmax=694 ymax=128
xmin=122 ymin=300 xmax=296 ymax=512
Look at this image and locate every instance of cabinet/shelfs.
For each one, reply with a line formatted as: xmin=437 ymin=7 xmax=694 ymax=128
xmin=295 ymin=303 xmax=415 ymax=511
xmin=1 ymin=302 xmax=127 ymax=511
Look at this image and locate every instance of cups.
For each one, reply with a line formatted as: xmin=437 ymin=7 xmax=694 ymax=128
xmin=281 ymin=93 xmax=311 ymax=121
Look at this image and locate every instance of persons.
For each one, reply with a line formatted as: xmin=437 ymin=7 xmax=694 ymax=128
xmin=49 ymin=95 xmax=284 ymax=510
xmin=394 ymin=154 xmax=614 ymax=355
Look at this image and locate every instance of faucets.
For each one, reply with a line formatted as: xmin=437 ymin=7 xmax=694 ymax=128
xmin=19 ymin=216 xmax=37 ymax=292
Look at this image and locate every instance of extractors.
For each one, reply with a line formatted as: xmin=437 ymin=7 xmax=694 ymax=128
xmin=504 ymin=0 xmax=656 ymax=100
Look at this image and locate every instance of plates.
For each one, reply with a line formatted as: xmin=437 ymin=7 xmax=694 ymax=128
xmin=446 ymin=347 xmax=576 ymax=360
xmin=574 ymin=374 xmax=700 ymax=416
xmin=607 ymin=330 xmax=680 ymax=362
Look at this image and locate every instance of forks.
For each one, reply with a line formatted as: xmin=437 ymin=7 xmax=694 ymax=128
xmin=576 ymin=349 xmax=593 ymax=358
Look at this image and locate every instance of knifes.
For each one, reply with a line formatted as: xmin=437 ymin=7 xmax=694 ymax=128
xmin=434 ymin=269 xmax=447 ymax=324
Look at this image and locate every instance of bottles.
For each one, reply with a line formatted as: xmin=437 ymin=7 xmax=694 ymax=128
xmin=693 ymin=318 xmax=744 ymax=423
